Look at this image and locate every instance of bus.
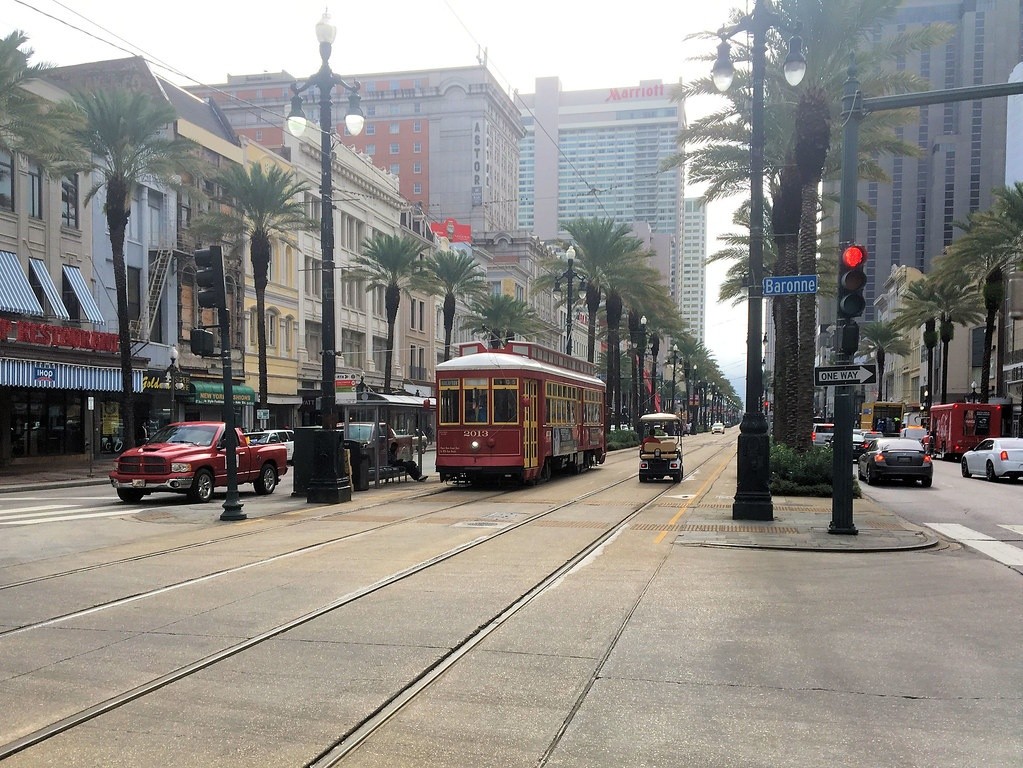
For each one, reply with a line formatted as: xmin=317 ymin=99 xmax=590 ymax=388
xmin=929 ymin=402 xmax=1001 ymax=461
xmin=434 ymin=340 xmax=607 ymax=488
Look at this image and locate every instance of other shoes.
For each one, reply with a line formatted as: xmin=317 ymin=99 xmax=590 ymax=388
xmin=418 ymin=475 xmax=428 ymax=482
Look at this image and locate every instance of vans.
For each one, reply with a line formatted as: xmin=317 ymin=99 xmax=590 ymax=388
xmin=900 ymin=426 xmax=927 ymax=443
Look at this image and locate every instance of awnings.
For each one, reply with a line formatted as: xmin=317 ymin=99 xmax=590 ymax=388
xmin=27 ymin=257 xmax=71 ymax=323
xmin=60 ymin=263 xmax=107 ymax=326
xmin=355 ymin=392 xmax=436 ymax=412
xmin=177 ymin=379 xmax=255 ymax=406
xmin=0 ymin=249 xmax=45 ymax=318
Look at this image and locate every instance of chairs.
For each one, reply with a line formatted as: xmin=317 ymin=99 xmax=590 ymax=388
xmin=363 ymin=448 xmax=410 ymax=487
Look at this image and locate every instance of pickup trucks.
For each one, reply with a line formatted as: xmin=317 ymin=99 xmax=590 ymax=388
xmin=107 ymin=421 xmax=289 ymax=504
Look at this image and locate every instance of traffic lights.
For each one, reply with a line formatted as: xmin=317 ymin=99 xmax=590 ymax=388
xmin=193 ymin=245 xmax=227 ymax=311
xmin=838 ymin=241 xmax=867 ymax=318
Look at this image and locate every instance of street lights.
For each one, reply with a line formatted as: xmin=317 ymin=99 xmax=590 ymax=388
xmin=971 ymin=380 xmax=978 ymax=405
xmin=551 ymin=246 xmax=587 ymax=357
xmin=629 ymin=316 xmax=654 ymax=421
xmin=283 ymin=5 xmax=366 ymax=506
xmin=690 ymin=363 xmax=739 ymax=435
xmin=710 ymin=0 xmax=807 ymax=522
xmin=170 ymin=344 xmax=180 ymax=424
xmin=666 ymin=343 xmax=684 ymax=414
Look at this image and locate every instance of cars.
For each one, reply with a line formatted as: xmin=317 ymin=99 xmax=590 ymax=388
xmin=338 ymin=422 xmax=397 ymax=469
xmin=961 ymin=437 xmax=1023 ymax=482
xmin=395 ymin=428 xmax=427 ymax=454
xmin=851 ymin=429 xmax=883 ymax=460
xmin=610 ymin=424 xmax=634 ymax=432
xmin=857 ymin=436 xmax=933 ymax=488
xmin=711 ymin=423 xmax=725 ymax=434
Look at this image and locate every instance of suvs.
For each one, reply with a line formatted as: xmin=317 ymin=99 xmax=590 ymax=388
xmin=243 ymin=430 xmax=295 ymax=467
xmin=809 ymin=423 xmax=835 ymax=448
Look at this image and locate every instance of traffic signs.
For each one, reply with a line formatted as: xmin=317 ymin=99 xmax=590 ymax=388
xmin=814 ymin=365 xmax=879 ymax=386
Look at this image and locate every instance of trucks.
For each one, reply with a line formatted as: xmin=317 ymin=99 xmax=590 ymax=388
xmin=860 ymin=401 xmax=904 ymax=437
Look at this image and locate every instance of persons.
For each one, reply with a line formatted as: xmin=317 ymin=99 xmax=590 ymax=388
xmin=387 ymin=441 xmax=429 ymax=481
xmin=241 ymin=425 xmax=264 ymax=441
xmin=388 ymin=424 xmax=397 ymax=438
xmin=686 ymin=422 xmax=690 ymax=436
xmin=135 ymin=421 xmax=149 ymax=446
xmin=464 ymin=394 xmax=503 ymax=422
xmin=280 ymin=423 xmax=289 ymax=442
xmin=641 ymin=428 xmax=660 ymax=450
